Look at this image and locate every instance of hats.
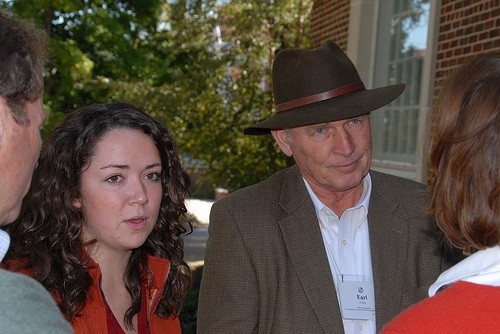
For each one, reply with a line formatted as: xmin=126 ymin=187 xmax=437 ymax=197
xmin=243 ymin=39 xmax=406 ymax=136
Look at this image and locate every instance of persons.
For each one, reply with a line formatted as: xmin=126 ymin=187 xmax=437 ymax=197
xmin=195 ymin=42 xmax=464 ymax=334
xmin=0 ymin=103 xmax=195 ymax=334
xmin=378 ymin=50 xmax=500 ymax=334
xmin=0 ymin=7 xmax=74 ymax=334
